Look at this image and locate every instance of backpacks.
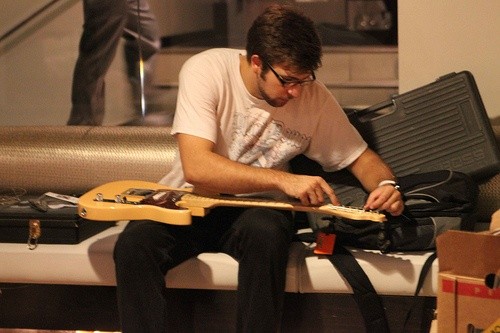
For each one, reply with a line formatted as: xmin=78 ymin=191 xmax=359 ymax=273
xmin=321 ymin=169 xmax=481 ymax=333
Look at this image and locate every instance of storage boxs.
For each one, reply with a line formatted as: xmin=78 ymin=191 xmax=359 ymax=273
xmin=435 ymin=230 xmax=500 ymax=333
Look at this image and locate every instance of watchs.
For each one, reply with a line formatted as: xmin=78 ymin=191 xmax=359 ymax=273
xmin=378 ymin=179 xmax=402 ymax=192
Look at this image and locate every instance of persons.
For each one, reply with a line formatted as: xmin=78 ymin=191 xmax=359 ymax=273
xmin=112 ymin=5 xmax=404 ymax=333
xmin=67 ymin=0 xmax=160 ymax=126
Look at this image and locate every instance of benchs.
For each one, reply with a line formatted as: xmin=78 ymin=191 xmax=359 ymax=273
xmin=0 ymin=124 xmax=500 ymax=330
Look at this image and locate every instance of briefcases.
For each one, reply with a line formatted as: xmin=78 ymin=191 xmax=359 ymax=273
xmin=306 ymin=69 xmax=500 ymax=232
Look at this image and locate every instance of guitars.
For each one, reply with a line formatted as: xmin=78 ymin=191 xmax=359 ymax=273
xmin=77 ymin=180 xmax=388 ymax=226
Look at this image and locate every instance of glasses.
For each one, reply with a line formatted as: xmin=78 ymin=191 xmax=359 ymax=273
xmin=266 ymin=62 xmax=316 ymax=87
